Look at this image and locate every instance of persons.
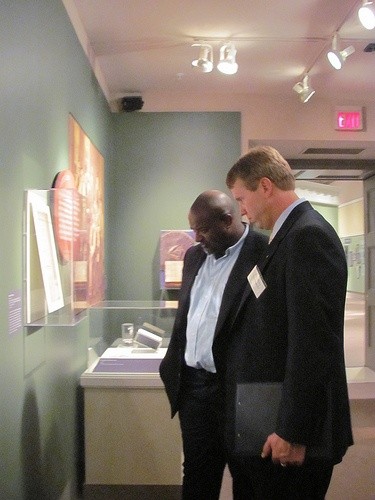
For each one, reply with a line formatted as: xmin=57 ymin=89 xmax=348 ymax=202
xmin=159 ymin=190 xmax=269 ymax=500
xmin=225 ymin=145 xmax=354 ymax=500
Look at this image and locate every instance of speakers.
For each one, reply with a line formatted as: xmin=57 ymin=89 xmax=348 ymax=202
xmin=121 ymin=95 xmax=144 ymax=112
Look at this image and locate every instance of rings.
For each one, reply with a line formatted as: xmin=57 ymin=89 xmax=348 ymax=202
xmin=280 ymin=464 xmax=287 ymax=467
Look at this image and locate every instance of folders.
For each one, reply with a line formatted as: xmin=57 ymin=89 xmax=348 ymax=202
xmin=234 ymin=380 xmax=332 ymax=460
